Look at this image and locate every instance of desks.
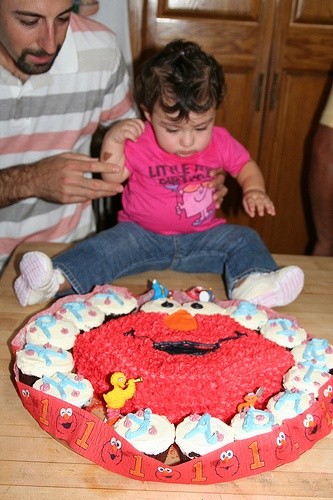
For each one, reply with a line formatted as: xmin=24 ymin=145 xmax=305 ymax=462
xmin=0 ymin=243 xmax=333 ymax=500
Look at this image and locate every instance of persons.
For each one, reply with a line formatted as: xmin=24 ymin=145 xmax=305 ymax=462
xmin=14 ymin=39 xmax=304 ymax=319
xmin=309 ymin=83 xmax=333 ymax=255
xmin=0 ymin=0 xmax=141 ymax=276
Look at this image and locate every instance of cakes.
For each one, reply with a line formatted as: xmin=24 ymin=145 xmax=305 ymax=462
xmin=15 ymin=278 xmax=333 ymax=466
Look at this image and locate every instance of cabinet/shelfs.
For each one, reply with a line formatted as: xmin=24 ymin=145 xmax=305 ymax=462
xmin=130 ymin=0 xmax=333 ymax=254
